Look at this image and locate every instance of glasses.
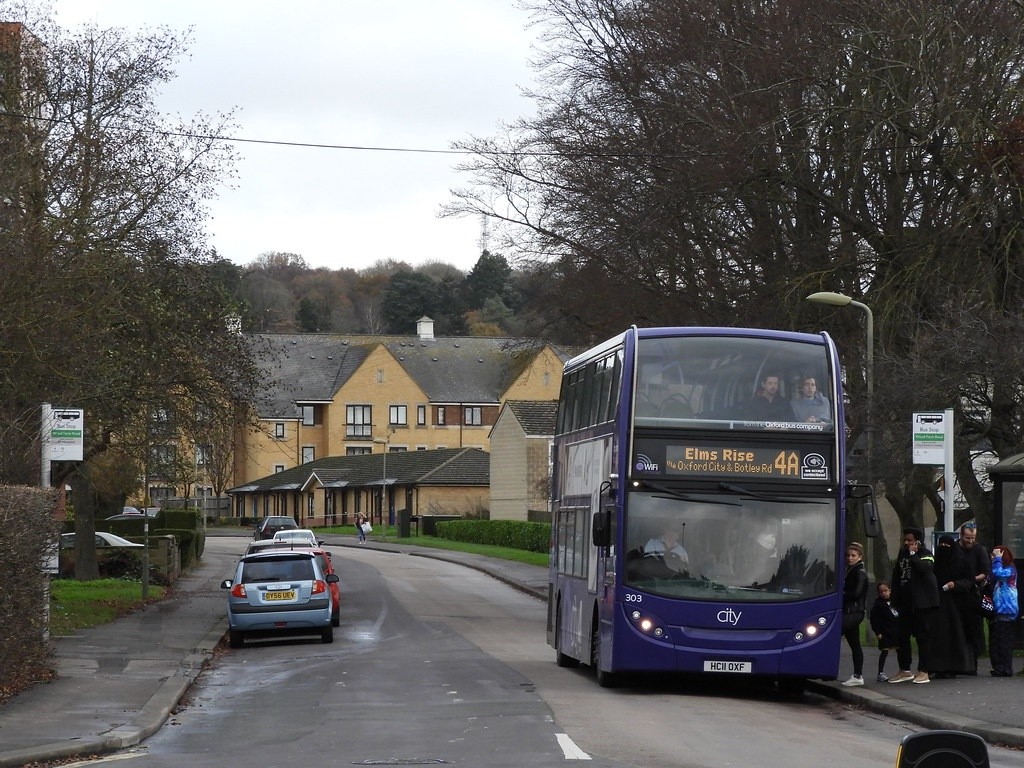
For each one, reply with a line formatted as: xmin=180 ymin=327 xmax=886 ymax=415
xmin=965 ymin=524 xmax=978 ymax=528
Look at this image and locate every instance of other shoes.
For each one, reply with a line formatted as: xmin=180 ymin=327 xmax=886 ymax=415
xmin=989 ymin=669 xmax=1013 ymax=677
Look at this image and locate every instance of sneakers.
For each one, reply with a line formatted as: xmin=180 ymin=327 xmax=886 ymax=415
xmin=877 ymin=672 xmax=890 ymax=682
xmin=888 ymin=670 xmax=914 ymax=684
xmin=842 ymin=674 xmax=865 ymax=686
xmin=912 ymin=671 xmax=930 ymax=684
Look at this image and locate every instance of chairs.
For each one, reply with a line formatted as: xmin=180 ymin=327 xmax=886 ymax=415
xmin=635 ymin=392 xmax=694 ymax=419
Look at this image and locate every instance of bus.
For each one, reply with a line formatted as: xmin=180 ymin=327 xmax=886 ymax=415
xmin=546 ymin=325 xmax=880 ymax=688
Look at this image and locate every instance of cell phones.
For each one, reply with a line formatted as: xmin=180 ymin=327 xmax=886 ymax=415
xmin=915 ymin=541 xmax=921 ymax=546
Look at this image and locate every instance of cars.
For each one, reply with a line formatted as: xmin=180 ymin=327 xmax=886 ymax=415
xmin=140 ymin=508 xmax=161 ymax=517
xmin=253 ymin=516 xmax=298 ymax=541
xmin=258 ymin=547 xmax=340 ymax=626
xmin=273 ymin=529 xmax=325 ymax=548
xmin=37 ymin=532 xmax=145 ymax=574
xmin=122 ymin=507 xmax=139 ymax=514
xmin=104 ymin=514 xmax=155 ymax=520
xmin=220 ymin=551 xmax=340 ymax=645
xmin=244 ymin=538 xmax=314 ymax=556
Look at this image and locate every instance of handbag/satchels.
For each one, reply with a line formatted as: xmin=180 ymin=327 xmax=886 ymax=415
xmin=843 ymin=607 xmax=867 ymax=625
xmin=361 ymin=521 xmax=372 ymax=534
xmin=978 ymin=581 xmax=995 ymax=616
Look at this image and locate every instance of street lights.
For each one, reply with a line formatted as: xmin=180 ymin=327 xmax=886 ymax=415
xmin=373 ymin=439 xmax=387 ymax=527
xmin=807 ymin=292 xmax=876 ymax=619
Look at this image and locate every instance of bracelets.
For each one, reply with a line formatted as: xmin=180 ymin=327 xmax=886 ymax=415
xmin=909 ymin=551 xmax=915 ymax=556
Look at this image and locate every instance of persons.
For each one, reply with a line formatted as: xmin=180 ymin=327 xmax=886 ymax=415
xmin=643 ymin=523 xmax=690 ymax=571
xmin=356 ymin=511 xmax=369 ymax=545
xmin=753 ymin=370 xmax=799 ymax=422
xmin=840 ymin=541 xmax=868 ymax=686
xmin=791 ymin=375 xmax=832 ymax=419
xmin=870 ymin=520 xmax=1024 ymax=683
xmin=736 ymin=533 xmax=775 ymax=579
